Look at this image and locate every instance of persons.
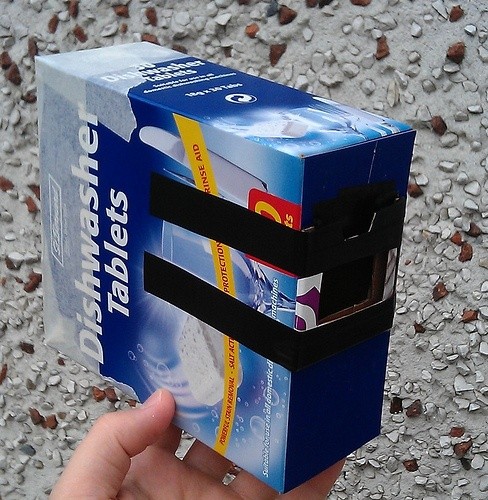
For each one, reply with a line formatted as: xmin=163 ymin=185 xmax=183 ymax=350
xmin=46 ymin=388 xmax=348 ymax=500
